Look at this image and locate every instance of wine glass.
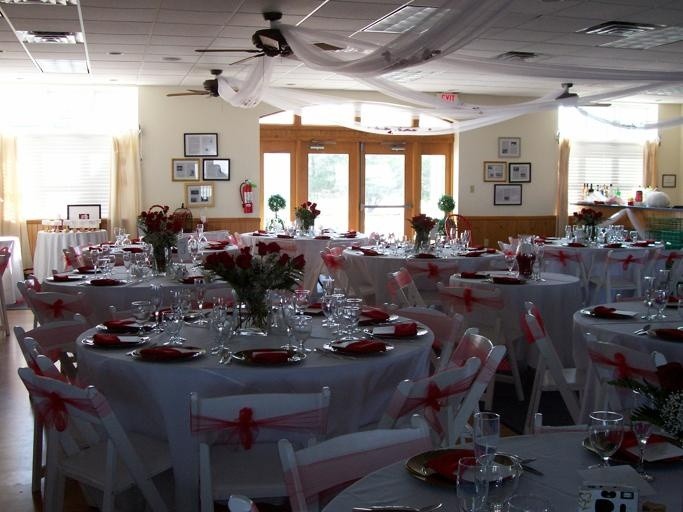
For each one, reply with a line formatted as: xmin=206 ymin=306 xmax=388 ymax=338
xmin=395 ymin=238 xmax=399 ymax=256
xmin=287 ymin=226 xmax=315 ymax=236
xmin=573 ymin=225 xmax=578 ymax=242
xmin=507 ymin=494 xmax=551 ymax=512
xmin=212 ymin=297 xmax=229 ymax=347
xmin=632 ymin=402 xmax=656 ymax=485
xmin=675 ymin=283 xmax=683 ymax=310
xmin=580 ymin=225 xmax=628 ymax=243
xmin=91 ymin=250 xmax=99 ymax=281
xmin=37 ymin=218 xmax=102 ymax=232
xmin=388 ymin=233 xmax=394 ymax=249
xmin=123 ymin=251 xmax=133 ymax=284
xmin=270 ymin=290 xmax=281 ymax=327
xmin=655 ymin=290 xmax=668 ymax=320
xmin=295 ymin=290 xmax=310 ymax=323
xmin=677 ymin=299 xmax=683 ymax=330
xmin=505 ymin=255 xmax=516 ymax=276
xmin=531 ymin=260 xmax=539 ymax=285
xmin=281 ymin=296 xmax=297 ymax=351
xmin=450 ymin=228 xmax=456 ymax=247
xmin=659 ymin=270 xmax=672 ymax=294
xmin=104 ymin=255 xmax=116 ymax=279
xmin=630 ymin=231 xmax=638 ymax=242
xmin=162 ymin=312 xmax=183 ymax=346
xmin=99 ymin=257 xmax=108 ymax=279
xmin=136 ymin=253 xmax=147 ymax=277
xmin=164 ymin=309 xmax=186 ymax=341
xmin=192 ymin=279 xmax=210 ymax=326
xmin=170 ymin=289 xmax=191 ymax=325
xmin=418 ymin=240 xmax=431 ymax=253
xmin=373 ymin=233 xmax=380 ymax=251
xmin=320 ymin=294 xmax=361 ymax=336
xmin=399 ymin=234 xmax=419 ymax=255
xmin=211 ymin=323 xmax=233 ymax=354
xmin=435 ymin=233 xmax=441 ymax=253
xmin=138 ymin=262 xmax=150 ymax=284
xmin=465 ymin=230 xmax=471 ymax=252
xmin=197 ymin=224 xmax=203 ymax=249
xmin=472 ymin=413 xmax=499 ymax=487
xmin=461 ymin=233 xmax=467 ymax=255
xmin=291 ymin=316 xmax=312 ymax=355
xmin=131 ymin=301 xmax=151 ymax=338
xmin=190 ymin=239 xmax=198 ymax=272
xmin=149 ymin=291 xmax=164 ymax=331
xmin=114 ymin=227 xmax=131 ymax=249
xmin=477 ymin=452 xmax=519 ymax=512
xmin=535 ymin=247 xmax=546 ymax=283
xmin=643 ymin=277 xmax=657 ymax=319
xmin=589 ymin=411 xmax=625 ymax=468
xmin=456 ymin=456 xmax=490 ymax=512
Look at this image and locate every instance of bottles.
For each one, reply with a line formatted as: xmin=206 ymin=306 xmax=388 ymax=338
xmin=582 ymin=183 xmax=622 ymax=197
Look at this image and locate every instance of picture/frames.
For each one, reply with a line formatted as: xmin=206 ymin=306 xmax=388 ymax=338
xmin=483 ymin=160 xmax=508 ymax=182
xmin=184 ymin=132 xmax=218 ymax=157
xmin=493 ymin=184 xmax=522 ymax=206
xmin=498 ymin=136 xmax=521 ymax=158
xmin=509 ymin=162 xmax=531 ymax=183
xmin=662 ymin=174 xmax=676 ymax=187
xmin=203 ymin=158 xmax=230 ymax=181
xmin=67 ymin=204 xmax=102 ymax=229
xmin=183 ymin=181 xmax=216 ymax=208
xmin=171 ymin=158 xmax=201 ymax=181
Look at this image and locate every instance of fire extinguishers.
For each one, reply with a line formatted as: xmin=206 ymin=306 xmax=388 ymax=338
xmin=240 ymin=179 xmax=253 ymax=213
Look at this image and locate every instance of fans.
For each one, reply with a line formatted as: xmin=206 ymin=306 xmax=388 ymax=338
xmin=554 ymin=82 xmax=613 ymax=107
xmin=166 ymin=69 xmax=241 ymax=96
xmin=194 ymin=11 xmax=344 ymax=66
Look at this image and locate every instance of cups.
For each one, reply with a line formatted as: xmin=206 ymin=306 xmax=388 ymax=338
xmin=636 ymin=191 xmax=642 ymax=201
xmin=322 ymin=279 xmax=335 ymax=294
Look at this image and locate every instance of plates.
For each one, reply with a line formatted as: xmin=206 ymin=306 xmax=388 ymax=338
xmin=580 ymin=308 xmax=638 ymax=322
xmin=296 ymin=302 xmax=328 ymax=315
xmin=649 ymin=295 xmax=682 ymax=308
xmin=81 ymin=335 xmax=152 ymax=348
xmin=95 ymin=320 xmax=159 ymax=331
xmin=629 ymin=241 xmax=655 ymax=247
xmin=126 ymin=248 xmax=147 ymax=253
xmin=456 ymin=271 xmax=490 ymax=279
xmin=600 ymin=244 xmax=626 ymax=247
xmin=650 ymin=329 xmax=682 ymax=340
xmin=207 ymin=240 xmax=233 ymax=245
xmin=584 ymin=430 xmax=680 ymax=467
xmin=46 ymin=275 xmax=84 ymax=282
xmin=131 ymin=237 xmax=145 ymax=244
xmin=405 ymin=447 xmax=513 ymax=485
xmin=323 ymin=341 xmax=396 ymax=356
xmin=358 ymin=309 xmax=399 ymax=323
xmin=461 ymin=252 xmax=485 ymax=257
xmin=180 ymin=276 xmax=214 ymax=286
xmin=73 ymin=266 xmax=101 ymax=273
xmin=563 ymin=244 xmax=586 ymax=247
xmin=126 ymin=346 xmax=206 ymax=362
xmin=411 ymin=254 xmax=441 ymax=259
xmin=565 ymin=226 xmax=571 ymax=242
xmin=234 ymin=348 xmax=306 ymax=365
xmin=541 ymin=234 xmax=561 ymax=241
xmin=491 ymin=277 xmax=527 ymax=286
xmin=84 ymin=279 xmax=127 ymax=286
xmin=204 ymin=245 xmax=229 ymax=250
xmin=482 ymin=249 xmax=496 ymax=253
xmin=467 ymin=245 xmax=484 ymax=250
xmin=535 ymin=239 xmax=553 ymax=244
xmin=364 ymin=325 xmax=428 ymax=339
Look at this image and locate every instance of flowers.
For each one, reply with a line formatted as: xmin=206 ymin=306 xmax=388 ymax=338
xmin=574 ymin=208 xmax=604 ymax=238
xmin=406 ymin=213 xmax=439 ymax=249
xmin=293 ymin=201 xmax=321 ymax=232
xmin=137 ymin=204 xmax=179 ymax=267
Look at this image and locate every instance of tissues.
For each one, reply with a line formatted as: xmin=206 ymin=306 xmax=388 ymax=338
xmin=573 ymin=464 xmax=655 ymax=512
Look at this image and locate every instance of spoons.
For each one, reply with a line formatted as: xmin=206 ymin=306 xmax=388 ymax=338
xmin=351 ymin=502 xmax=446 ymax=512
